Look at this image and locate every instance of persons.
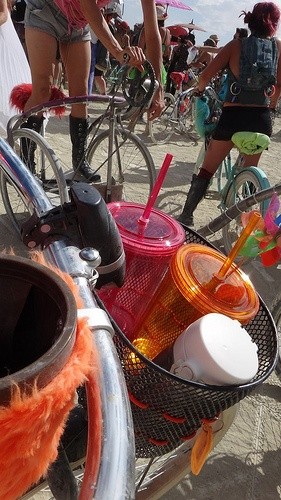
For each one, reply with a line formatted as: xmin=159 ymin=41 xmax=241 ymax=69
xmin=176 ymin=0 xmax=281 ymax=225
xmin=0 ymin=0 xmax=249 ymax=182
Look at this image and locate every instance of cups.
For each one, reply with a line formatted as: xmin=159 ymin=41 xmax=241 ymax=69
xmin=122 ymin=243 xmax=261 ymax=379
xmin=94 ymin=200 xmax=186 ymax=342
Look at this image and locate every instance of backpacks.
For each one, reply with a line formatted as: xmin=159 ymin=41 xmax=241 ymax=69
xmin=227 ymin=36 xmax=276 ymax=91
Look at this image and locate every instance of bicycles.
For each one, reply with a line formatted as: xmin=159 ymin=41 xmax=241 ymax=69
xmin=0 ymin=31 xmax=281 ymax=500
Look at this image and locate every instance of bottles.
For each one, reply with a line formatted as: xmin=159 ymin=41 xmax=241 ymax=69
xmin=180 ymin=98 xmax=190 ymax=113
xmin=121 ymin=312 xmax=259 ymax=446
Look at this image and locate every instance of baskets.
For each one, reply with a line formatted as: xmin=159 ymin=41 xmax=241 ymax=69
xmin=93 ymin=212 xmax=278 ymax=459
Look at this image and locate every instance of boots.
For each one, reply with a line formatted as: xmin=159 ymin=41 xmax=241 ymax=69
xmin=236 ymin=181 xmax=256 ymax=226
xmin=177 ymin=174 xmax=212 ymax=226
xmin=69 ymin=115 xmax=101 ymax=183
xmin=20 ymin=116 xmax=45 ymax=187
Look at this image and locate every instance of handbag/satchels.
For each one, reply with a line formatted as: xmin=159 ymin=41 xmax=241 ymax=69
xmin=180 ymin=69 xmax=198 ymax=90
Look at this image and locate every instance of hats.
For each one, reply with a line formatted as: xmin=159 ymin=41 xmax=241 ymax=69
xmin=156 ymin=6 xmax=168 ymax=20
xmin=210 ymin=35 xmax=219 ymax=41
xmin=103 ymin=8 xmax=117 ymax=18
xmin=118 ymin=21 xmax=128 ymax=32
